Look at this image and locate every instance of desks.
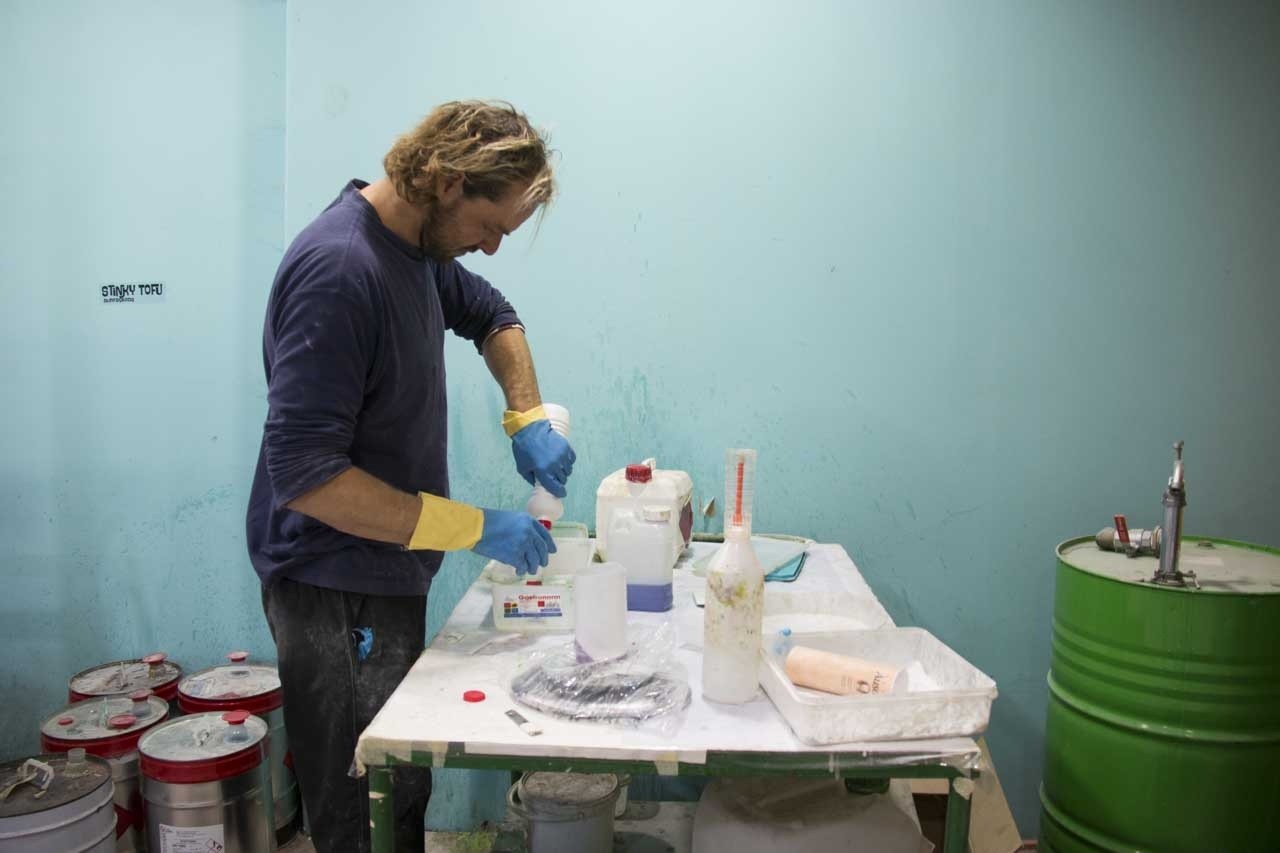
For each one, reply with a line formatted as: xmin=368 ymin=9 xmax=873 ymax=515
xmin=348 ymin=539 xmax=981 ymax=853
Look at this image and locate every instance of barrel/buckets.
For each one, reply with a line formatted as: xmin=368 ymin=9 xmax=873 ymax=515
xmin=692 ymin=774 xmax=921 ymax=853
xmin=1037 ymin=535 xmax=1280 ymax=853
xmin=137 ymin=710 xmax=278 ymax=853
xmin=0 ymin=748 xmax=118 ymax=853
xmin=41 ymin=688 xmax=170 ymax=853
xmin=65 ymin=653 xmax=182 ymax=719
xmin=505 ymin=769 xmax=633 ymax=853
xmin=175 ymin=652 xmax=300 ymax=850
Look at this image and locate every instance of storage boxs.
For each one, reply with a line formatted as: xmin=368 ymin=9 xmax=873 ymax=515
xmin=890 ymin=736 xmax=1025 ymax=853
xmin=493 ymin=522 xmax=598 ymax=632
xmin=760 ymin=626 xmax=998 ymax=744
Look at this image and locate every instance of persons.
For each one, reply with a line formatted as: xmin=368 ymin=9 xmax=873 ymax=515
xmin=242 ymin=99 xmax=579 ymax=853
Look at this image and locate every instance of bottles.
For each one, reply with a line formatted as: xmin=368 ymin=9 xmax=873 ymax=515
xmin=527 ymin=475 xmax=562 ymax=529
xmin=702 ymin=528 xmax=764 ymax=703
xmin=596 ymin=459 xmax=694 ymax=566
xmin=604 ymin=504 xmax=675 ymax=611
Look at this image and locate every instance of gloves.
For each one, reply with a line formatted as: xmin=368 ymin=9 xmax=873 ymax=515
xmin=503 ymin=407 xmax=577 ymax=497
xmin=402 ymin=491 xmax=557 ymax=576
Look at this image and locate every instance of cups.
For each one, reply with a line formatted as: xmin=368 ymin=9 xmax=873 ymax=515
xmin=574 ymin=562 xmax=629 ymax=666
